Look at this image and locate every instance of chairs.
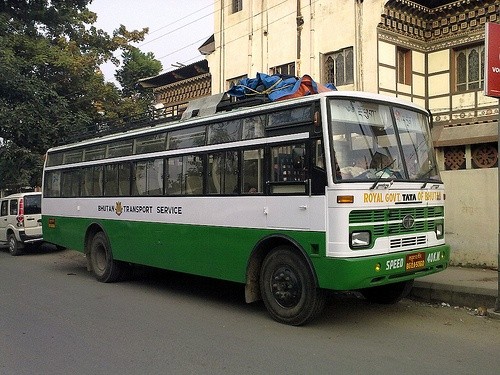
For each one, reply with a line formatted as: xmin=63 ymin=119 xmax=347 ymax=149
xmin=63 ymin=158 xmax=238 ymax=194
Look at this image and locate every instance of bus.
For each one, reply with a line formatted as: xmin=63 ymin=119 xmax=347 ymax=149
xmin=40 ymin=69 xmax=453 ymax=327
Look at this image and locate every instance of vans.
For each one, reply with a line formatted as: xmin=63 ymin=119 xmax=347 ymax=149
xmin=0 ymin=191 xmax=68 ymax=257
